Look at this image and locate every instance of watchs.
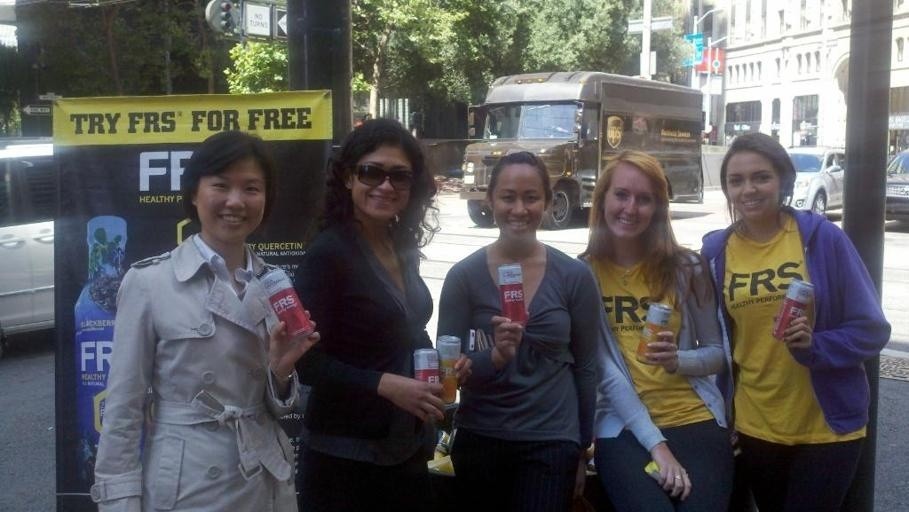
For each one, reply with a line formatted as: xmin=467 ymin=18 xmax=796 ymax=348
xmin=268 ymin=366 xmax=294 ymax=386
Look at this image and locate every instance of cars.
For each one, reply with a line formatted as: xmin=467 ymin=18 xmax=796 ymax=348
xmin=885 ymin=148 xmax=909 ymax=225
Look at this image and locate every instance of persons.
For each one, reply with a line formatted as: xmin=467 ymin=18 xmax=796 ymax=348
xmin=577 ymin=152 xmax=738 ymax=510
xmin=93 ymin=128 xmax=320 ymax=511
xmin=438 ymin=152 xmax=605 ymax=510
xmin=289 ymin=119 xmax=475 ymax=507
xmin=701 ymin=132 xmax=891 ymax=509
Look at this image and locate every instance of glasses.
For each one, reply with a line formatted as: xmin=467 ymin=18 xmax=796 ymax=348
xmin=351 ymin=164 xmax=417 ymax=191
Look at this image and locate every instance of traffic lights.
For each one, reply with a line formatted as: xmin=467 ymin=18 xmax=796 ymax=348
xmin=219 ymin=1 xmax=231 ymax=31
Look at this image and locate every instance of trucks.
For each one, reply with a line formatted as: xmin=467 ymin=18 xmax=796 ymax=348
xmin=458 ymin=71 xmax=706 ymax=230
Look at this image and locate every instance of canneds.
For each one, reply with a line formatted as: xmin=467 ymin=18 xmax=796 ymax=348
xmin=436 ymin=335 xmax=461 ymax=404
xmin=772 ymin=278 xmax=814 ymax=342
xmin=635 ymin=302 xmax=672 ymax=365
xmin=260 ymin=268 xmax=314 ymax=345
xmin=497 ymin=263 xmax=528 ymax=327
xmin=413 ymin=347 xmax=440 ymax=385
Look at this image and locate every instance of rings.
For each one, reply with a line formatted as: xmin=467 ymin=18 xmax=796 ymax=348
xmin=672 ymin=474 xmax=683 ymax=482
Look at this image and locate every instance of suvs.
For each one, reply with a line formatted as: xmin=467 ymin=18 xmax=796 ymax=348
xmin=778 ymin=142 xmax=845 ymax=220
xmin=1 ymin=133 xmax=56 ymax=339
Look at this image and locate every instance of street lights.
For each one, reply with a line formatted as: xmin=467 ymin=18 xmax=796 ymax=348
xmin=704 ymin=29 xmax=755 ymax=136
xmin=690 ymin=4 xmax=729 ymax=92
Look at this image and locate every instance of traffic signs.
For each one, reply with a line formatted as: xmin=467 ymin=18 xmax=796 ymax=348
xmin=275 ymin=6 xmax=287 ymax=36
